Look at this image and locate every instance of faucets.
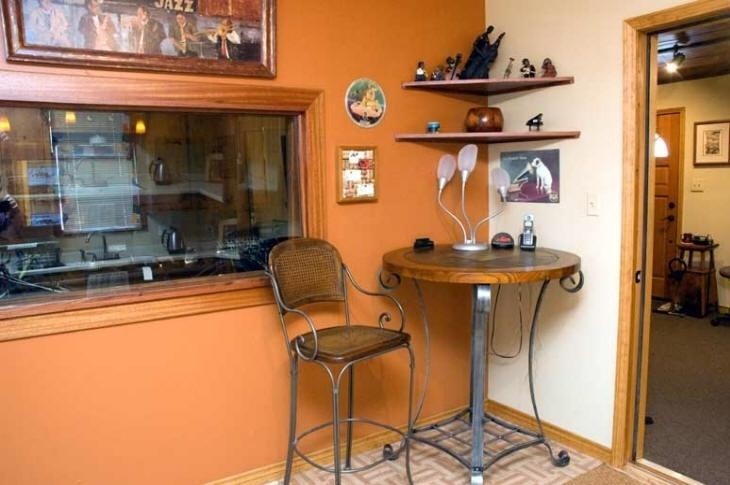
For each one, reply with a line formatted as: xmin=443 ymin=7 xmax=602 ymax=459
xmin=86 ymin=230 xmax=108 ymax=255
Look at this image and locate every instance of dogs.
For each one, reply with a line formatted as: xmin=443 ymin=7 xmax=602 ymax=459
xmin=531 ymin=158 xmax=553 ymax=190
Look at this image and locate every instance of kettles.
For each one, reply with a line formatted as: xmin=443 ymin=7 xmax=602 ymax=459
xmin=161 ymin=226 xmax=186 ymax=255
xmin=149 ymin=155 xmax=170 ymax=185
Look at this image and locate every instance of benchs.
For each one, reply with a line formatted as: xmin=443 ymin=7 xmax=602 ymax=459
xmin=671 ymin=242 xmax=720 ymax=318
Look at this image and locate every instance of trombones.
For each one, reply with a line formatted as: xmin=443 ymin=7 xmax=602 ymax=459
xmin=196 ymin=25 xmax=239 ymax=37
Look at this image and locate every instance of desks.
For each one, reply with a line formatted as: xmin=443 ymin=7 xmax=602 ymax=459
xmin=381 ymin=240 xmax=585 ymax=484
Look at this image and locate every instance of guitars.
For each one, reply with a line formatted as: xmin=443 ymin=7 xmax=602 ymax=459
xmin=35 ymin=21 xmax=66 ymax=44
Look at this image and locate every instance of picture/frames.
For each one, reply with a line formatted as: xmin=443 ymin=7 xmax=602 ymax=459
xmin=0 ymin=0 xmax=280 ymax=81
xmin=336 ymin=144 xmax=380 ymax=205
xmin=691 ymin=120 xmax=730 ymax=168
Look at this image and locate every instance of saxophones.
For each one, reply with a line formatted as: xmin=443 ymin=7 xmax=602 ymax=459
xmin=94 ymin=8 xmax=110 ymax=52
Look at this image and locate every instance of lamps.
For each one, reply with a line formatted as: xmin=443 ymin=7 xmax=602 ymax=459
xmin=665 ymin=43 xmax=689 ymax=74
xmin=437 ymin=144 xmax=514 ymax=255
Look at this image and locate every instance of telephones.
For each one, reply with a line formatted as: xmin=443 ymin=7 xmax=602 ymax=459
xmin=519 ymin=213 xmax=536 ymax=250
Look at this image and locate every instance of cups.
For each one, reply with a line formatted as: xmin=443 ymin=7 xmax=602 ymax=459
xmin=684 ymin=233 xmax=692 ymax=243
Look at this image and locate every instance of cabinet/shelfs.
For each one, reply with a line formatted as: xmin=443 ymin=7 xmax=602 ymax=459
xmin=395 ymin=74 xmax=581 ymax=146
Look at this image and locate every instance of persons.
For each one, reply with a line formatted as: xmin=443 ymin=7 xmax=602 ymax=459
xmin=206 ymin=16 xmax=242 ymax=63
xmin=415 ymin=24 xmax=557 ymax=80
xmin=167 ymin=9 xmax=200 ymax=60
xmin=75 ymin=0 xmax=122 ymax=52
xmin=28 ymin=0 xmax=72 ymax=45
xmin=127 ymin=4 xmax=167 ymax=57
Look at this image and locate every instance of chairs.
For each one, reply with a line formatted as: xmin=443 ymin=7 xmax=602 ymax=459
xmin=265 ymin=235 xmax=422 ymax=485
